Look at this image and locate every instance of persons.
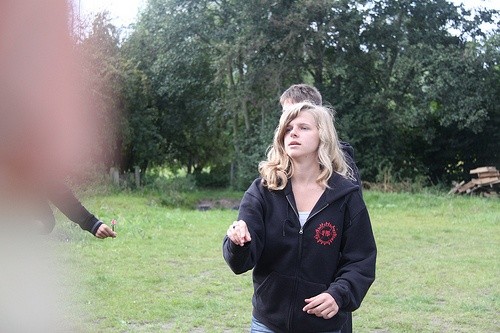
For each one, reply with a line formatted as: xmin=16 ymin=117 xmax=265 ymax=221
xmin=34 ymin=168 xmax=117 ymax=240
xmin=222 ymin=99 xmax=377 ymax=333
xmin=279 ymin=84 xmax=364 ymax=200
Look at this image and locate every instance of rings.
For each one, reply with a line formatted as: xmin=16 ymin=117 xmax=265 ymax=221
xmin=233 ymin=225 xmax=239 ymax=230
